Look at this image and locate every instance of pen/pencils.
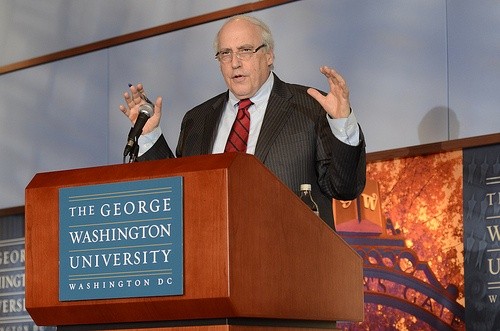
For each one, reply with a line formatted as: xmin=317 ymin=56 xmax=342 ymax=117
xmin=128 ymin=83 xmax=154 ymax=107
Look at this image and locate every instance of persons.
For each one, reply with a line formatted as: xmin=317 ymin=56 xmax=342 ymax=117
xmin=119 ymin=15 xmax=367 ymax=231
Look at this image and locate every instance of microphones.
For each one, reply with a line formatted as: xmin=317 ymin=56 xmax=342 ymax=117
xmin=123 ymin=103 xmax=154 ymax=156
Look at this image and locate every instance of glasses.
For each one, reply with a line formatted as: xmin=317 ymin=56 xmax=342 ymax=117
xmin=215 ymin=44 xmax=267 ymax=63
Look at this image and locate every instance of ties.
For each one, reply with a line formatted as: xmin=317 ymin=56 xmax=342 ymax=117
xmin=224 ymin=99 xmax=252 ymax=153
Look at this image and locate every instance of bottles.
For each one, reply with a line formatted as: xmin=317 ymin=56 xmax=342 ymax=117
xmin=300 ymin=184 xmax=319 ymax=217
